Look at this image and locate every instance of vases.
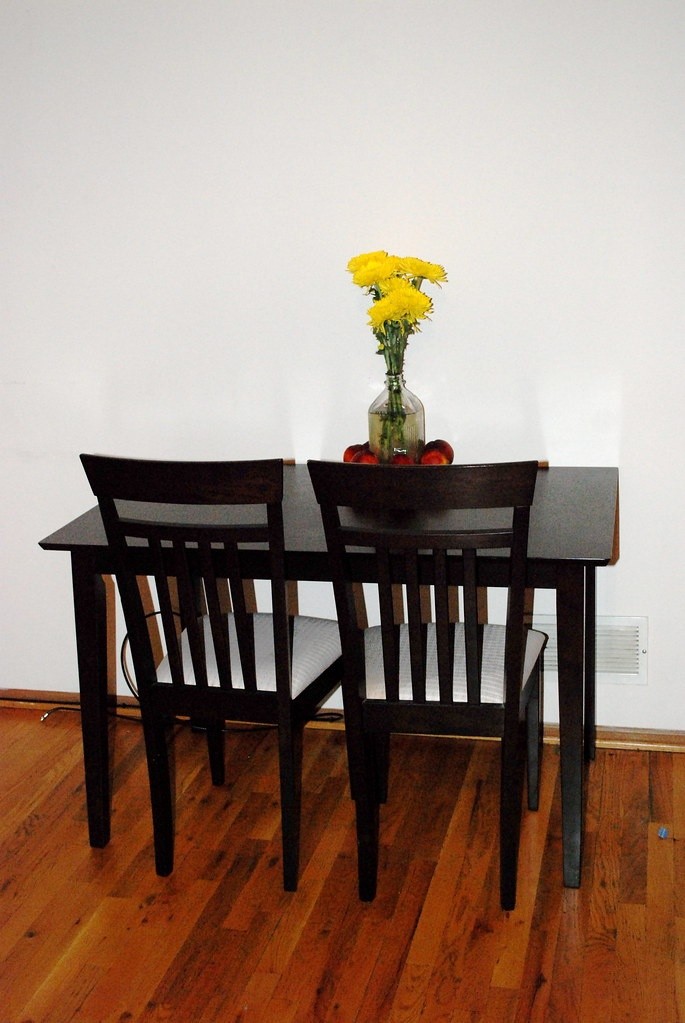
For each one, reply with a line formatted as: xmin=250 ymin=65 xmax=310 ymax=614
xmin=368 ymin=373 xmax=427 ymax=461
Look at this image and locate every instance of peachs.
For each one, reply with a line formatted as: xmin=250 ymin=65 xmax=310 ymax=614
xmin=343 ymin=439 xmax=454 ymax=465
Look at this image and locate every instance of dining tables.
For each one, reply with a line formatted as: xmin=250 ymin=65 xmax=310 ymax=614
xmin=38 ymin=467 xmax=620 ymax=888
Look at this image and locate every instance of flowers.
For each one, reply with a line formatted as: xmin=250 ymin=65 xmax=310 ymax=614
xmin=346 ymin=250 xmax=449 ymax=461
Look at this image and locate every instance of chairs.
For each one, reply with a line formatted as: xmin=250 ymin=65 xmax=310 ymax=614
xmin=79 ymin=453 xmax=342 ymax=891
xmin=305 ymin=460 xmax=550 ymax=911
